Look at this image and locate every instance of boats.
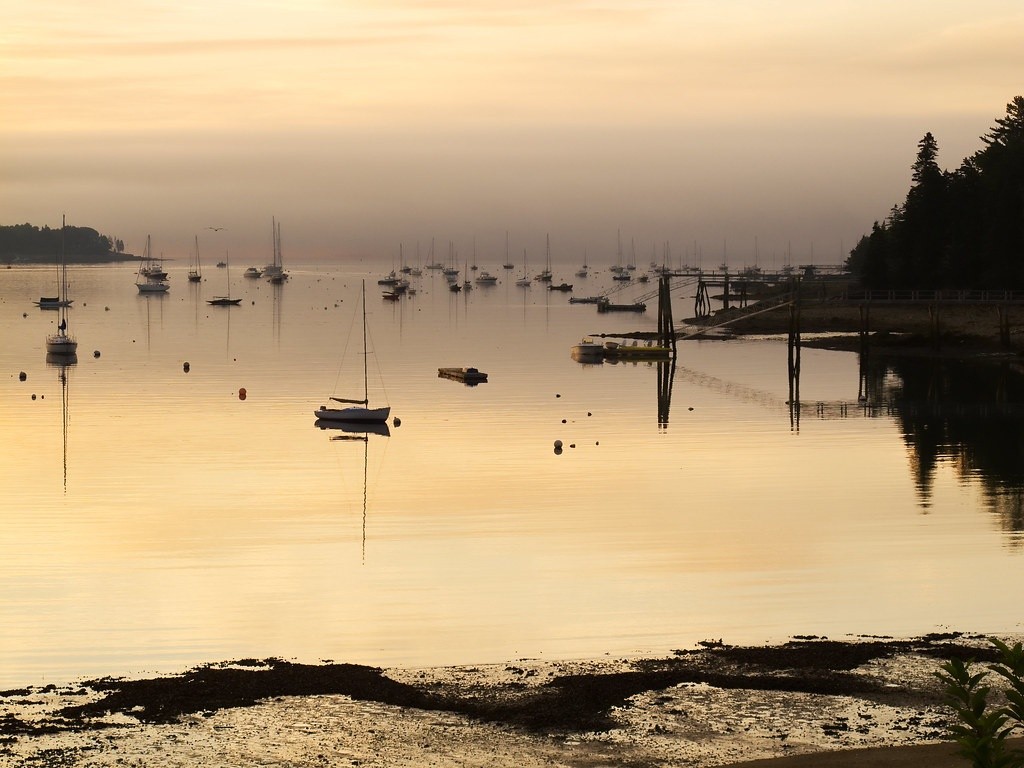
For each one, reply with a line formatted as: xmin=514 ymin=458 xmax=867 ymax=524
xmin=575 ymin=247 xmax=588 ymax=277
xmin=449 ymin=284 xmax=462 ymax=291
xmin=547 ymin=283 xmax=573 ymax=290
xmin=438 ymin=366 xmax=490 ymax=380
xmin=637 ymin=274 xmax=649 ymax=282
xmin=605 ymin=341 xmax=673 ymax=355
xmin=572 ymin=338 xmax=604 ymax=354
xmin=475 ymin=271 xmax=498 ymax=283
xmin=216 ymin=261 xmax=226 ymax=267
xmin=597 ymin=298 xmax=647 ymax=311
xmin=244 ymin=267 xmax=262 ymax=277
xmin=567 ymin=295 xmax=609 ymax=304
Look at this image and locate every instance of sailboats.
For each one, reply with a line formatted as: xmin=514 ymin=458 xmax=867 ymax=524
xmin=187 ymin=235 xmax=202 ymax=281
xmin=377 ymin=236 xmax=421 ymax=299
xmin=315 ymin=278 xmax=391 ymax=423
xmin=627 ymin=238 xmax=637 ymax=269
xmin=514 ymin=247 xmax=532 ymax=286
xmin=502 ymin=229 xmax=515 ymax=270
xmin=738 ymin=235 xmax=845 ymax=279
xmin=532 ymin=232 xmax=553 ymax=282
xmin=443 ymin=239 xmax=460 ymax=282
xmin=609 ymin=228 xmax=624 ymax=271
xmin=43 ymin=240 xmax=78 ymax=354
xmin=314 ymin=419 xmax=391 ymax=567
xmin=260 ymin=215 xmax=289 ymax=284
xmin=46 ymin=355 xmax=78 ymax=492
xmin=613 ymin=240 xmax=632 ymax=280
xmin=205 ymin=248 xmax=243 ymax=305
xmin=31 ymin=212 xmax=74 ymax=306
xmin=651 ymin=239 xmax=702 ymax=275
xmin=463 ymin=258 xmax=472 ymax=288
xmin=423 ymin=234 xmax=444 ymax=269
xmin=133 ymin=233 xmax=171 ymax=292
xmin=719 ymin=240 xmax=728 ymax=270
